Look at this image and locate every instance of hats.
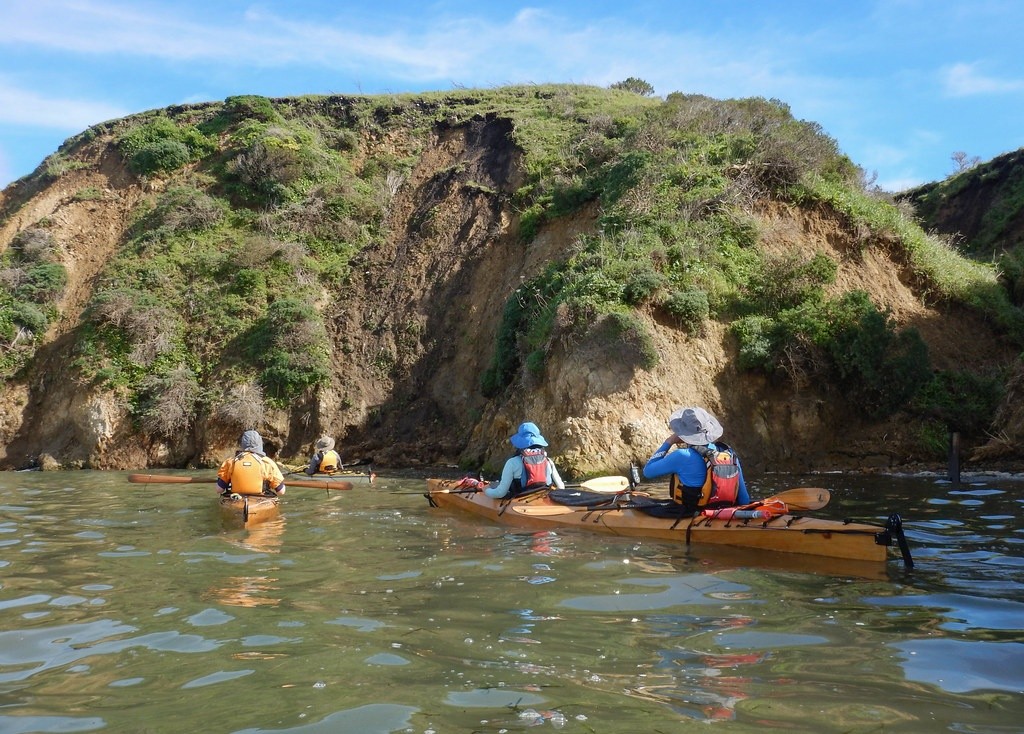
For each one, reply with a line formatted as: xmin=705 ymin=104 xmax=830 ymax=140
xmin=236 ymin=430 xmax=266 ymax=455
xmin=670 ymin=407 xmax=724 ymax=446
xmin=317 ymin=436 xmax=335 ymax=451
xmin=510 ymin=422 xmax=548 ymax=448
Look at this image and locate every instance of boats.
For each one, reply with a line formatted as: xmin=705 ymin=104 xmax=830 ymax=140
xmin=276 ymin=461 xmax=376 ymax=486
xmin=427 ymin=478 xmax=914 ymax=570
xmin=220 ymin=492 xmax=281 ymax=514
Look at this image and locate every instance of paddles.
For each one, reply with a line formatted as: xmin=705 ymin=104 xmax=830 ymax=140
xmin=390 ymin=488 xmax=482 ymax=493
xmin=285 ymin=457 xmax=374 ymax=475
xmin=128 ymin=473 xmax=353 ymax=490
xmin=586 ymin=487 xmax=830 ymax=512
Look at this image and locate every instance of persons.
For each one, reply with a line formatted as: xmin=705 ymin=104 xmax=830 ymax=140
xmin=643 ymin=408 xmax=750 ymax=509
xmin=302 ymin=436 xmax=343 ymax=475
xmin=482 ymin=423 xmax=566 ymax=499
xmin=217 ymin=430 xmax=285 ymax=496
